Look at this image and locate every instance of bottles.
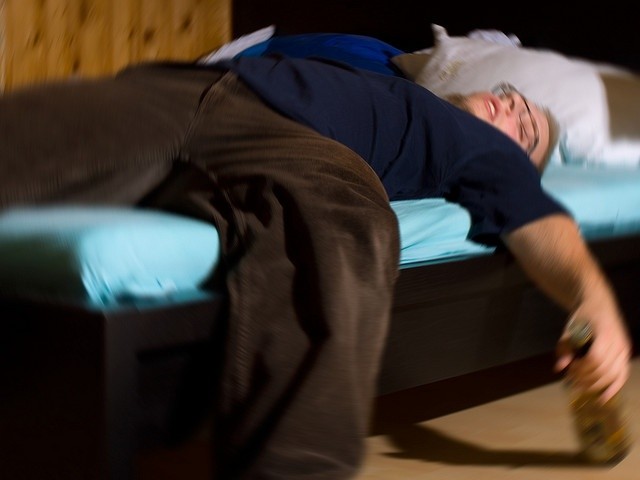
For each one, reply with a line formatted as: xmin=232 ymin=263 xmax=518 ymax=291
xmin=564 ymin=315 xmax=632 ymax=465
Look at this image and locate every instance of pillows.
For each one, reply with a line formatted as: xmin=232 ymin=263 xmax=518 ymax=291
xmin=414 ymin=25 xmax=640 ymax=163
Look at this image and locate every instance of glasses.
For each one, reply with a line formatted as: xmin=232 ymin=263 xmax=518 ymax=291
xmin=491 ymin=82 xmax=539 ymax=158
